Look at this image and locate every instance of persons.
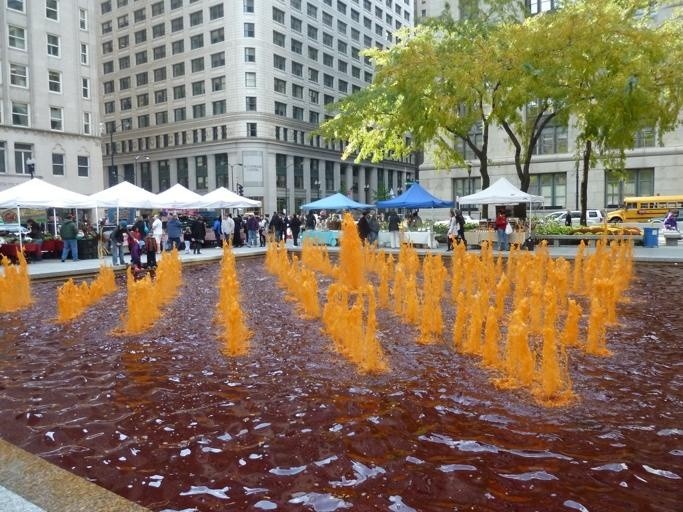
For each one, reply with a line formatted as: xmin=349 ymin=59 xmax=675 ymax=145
xmin=23 ymin=209 xmax=422 ymax=274
xmin=663 ymin=209 xmax=680 ymax=234
xmin=446 ymin=210 xmax=458 ymax=250
xmin=564 ymin=210 xmax=573 ymax=226
xmin=497 ymin=210 xmax=510 ymax=252
xmin=457 ymin=210 xmax=468 ymax=252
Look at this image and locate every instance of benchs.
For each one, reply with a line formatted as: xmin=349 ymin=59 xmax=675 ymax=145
xmin=664 ymin=232 xmax=681 ymax=246
xmin=536 ymin=235 xmax=643 ymax=248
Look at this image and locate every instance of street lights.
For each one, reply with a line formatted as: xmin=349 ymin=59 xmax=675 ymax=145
xmin=315 ymin=180 xmax=320 ymax=201
xmin=364 ymin=184 xmax=370 ymax=204
xmin=221 ymin=161 xmax=243 ymax=215
xmin=110 ymin=123 xmax=129 ymax=187
xmin=134 ymin=154 xmax=150 ymax=188
xmin=27 ymin=158 xmax=36 ymax=179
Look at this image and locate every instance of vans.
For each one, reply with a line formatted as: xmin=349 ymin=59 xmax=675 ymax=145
xmin=537 ymin=210 xmax=604 ymax=227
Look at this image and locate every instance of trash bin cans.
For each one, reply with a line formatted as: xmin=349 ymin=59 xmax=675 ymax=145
xmin=643 ymin=228 xmax=659 ymax=248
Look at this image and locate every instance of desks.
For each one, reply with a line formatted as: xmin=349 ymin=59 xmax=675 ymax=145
xmin=302 ymin=231 xmax=528 ymax=249
xmin=0 ymin=229 xmax=247 ymax=265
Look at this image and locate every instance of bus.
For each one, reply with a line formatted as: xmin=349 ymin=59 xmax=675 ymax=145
xmin=605 ymin=196 xmax=683 ymax=224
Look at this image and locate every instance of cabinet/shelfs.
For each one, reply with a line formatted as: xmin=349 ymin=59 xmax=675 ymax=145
xmin=479 ymin=221 xmax=515 ymax=231
